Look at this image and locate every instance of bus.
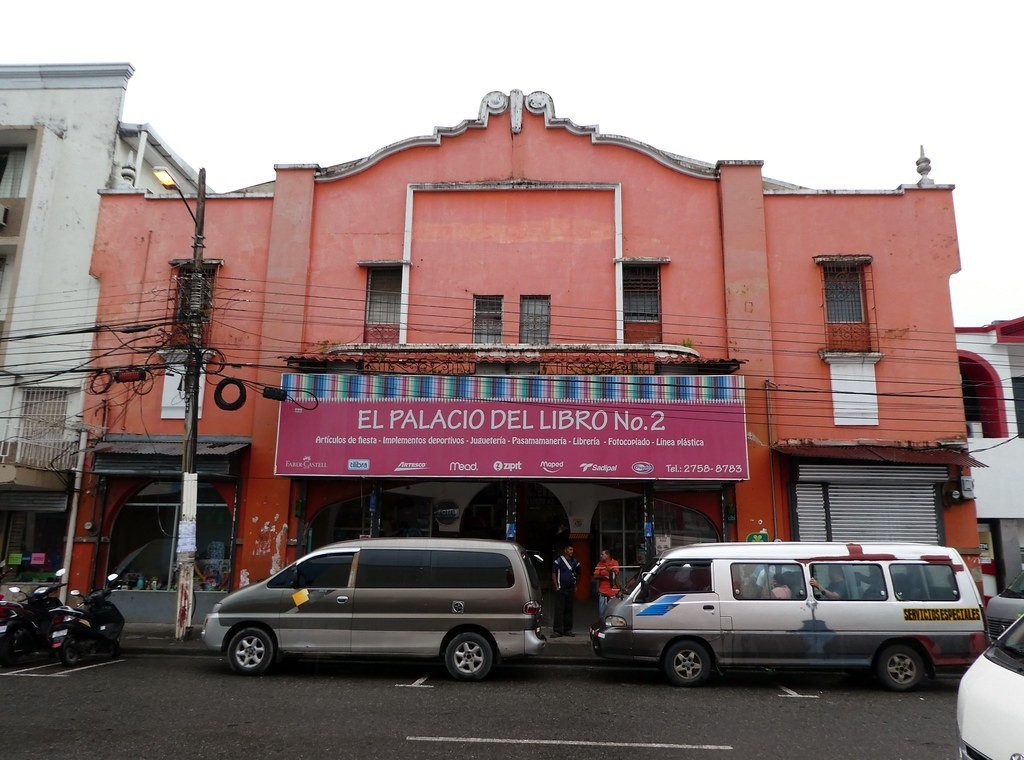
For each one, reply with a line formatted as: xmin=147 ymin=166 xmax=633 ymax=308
xmin=590 ymin=540 xmax=992 ymax=692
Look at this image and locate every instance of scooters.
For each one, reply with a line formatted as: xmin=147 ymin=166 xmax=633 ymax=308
xmin=0 ymin=568 xmax=69 ymax=664
xmin=48 ymin=573 xmax=125 ymax=666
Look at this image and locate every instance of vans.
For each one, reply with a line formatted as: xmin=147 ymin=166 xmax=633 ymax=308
xmin=201 ymin=537 xmax=547 ymax=682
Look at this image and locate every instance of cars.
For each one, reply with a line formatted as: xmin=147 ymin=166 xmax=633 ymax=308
xmin=956 ymin=614 xmax=1024 ymax=760
xmin=987 ymin=568 xmax=1024 ymax=640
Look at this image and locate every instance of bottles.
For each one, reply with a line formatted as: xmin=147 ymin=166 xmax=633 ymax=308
xmin=137 ymin=577 xmax=144 ymax=590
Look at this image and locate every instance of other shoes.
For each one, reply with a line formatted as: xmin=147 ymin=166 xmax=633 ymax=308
xmin=563 ymin=631 xmax=575 ymax=637
xmin=550 ymin=632 xmax=562 ymax=638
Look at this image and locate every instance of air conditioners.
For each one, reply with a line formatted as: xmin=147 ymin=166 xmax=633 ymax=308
xmin=967 ymin=422 xmax=983 ymax=437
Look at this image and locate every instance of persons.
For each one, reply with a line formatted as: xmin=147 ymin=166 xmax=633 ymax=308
xmin=750 ymin=564 xmax=934 ymax=601
xmin=549 ymin=544 xmax=581 ymax=638
xmin=594 ymin=549 xmax=619 ymax=621
xmin=193 ymin=551 xmax=204 ymax=579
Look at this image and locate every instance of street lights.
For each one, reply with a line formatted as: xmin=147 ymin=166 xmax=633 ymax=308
xmin=152 ymin=165 xmax=206 ymax=638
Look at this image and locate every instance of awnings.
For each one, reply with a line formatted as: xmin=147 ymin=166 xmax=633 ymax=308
xmin=81 ymin=439 xmax=250 ymax=455
